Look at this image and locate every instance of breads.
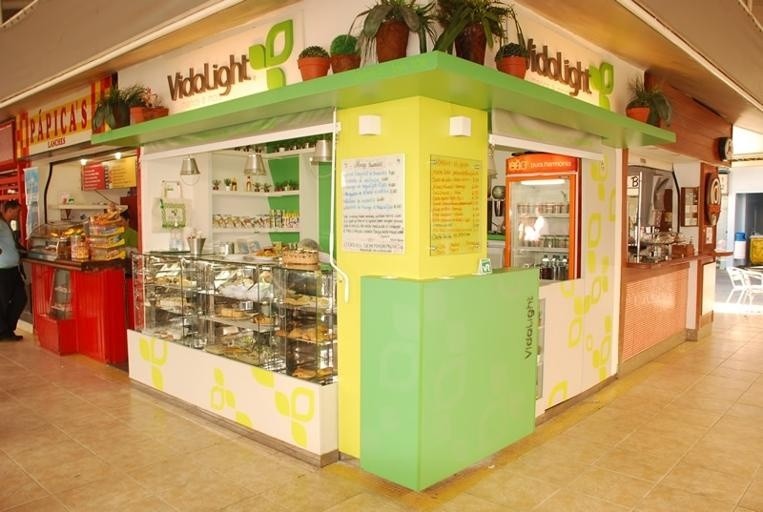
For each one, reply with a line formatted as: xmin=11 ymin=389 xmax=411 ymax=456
xmin=155 ymin=275 xmax=336 ymax=379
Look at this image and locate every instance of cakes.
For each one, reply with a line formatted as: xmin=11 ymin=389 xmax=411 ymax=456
xmin=283 ymin=249 xmax=318 ymax=265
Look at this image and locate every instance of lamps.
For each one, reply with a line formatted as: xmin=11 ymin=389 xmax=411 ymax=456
xmin=309 ymin=133 xmax=332 ymax=180
xmin=180 ymin=153 xmax=200 ymax=187
xmin=244 ymin=146 xmax=267 ymax=175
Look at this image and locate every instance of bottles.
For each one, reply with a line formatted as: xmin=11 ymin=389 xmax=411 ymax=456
xmin=539 ymin=254 xmax=569 ymax=280
xmin=231 ymin=177 xmax=238 ymax=191
xmin=246 ymin=176 xmax=252 ymax=191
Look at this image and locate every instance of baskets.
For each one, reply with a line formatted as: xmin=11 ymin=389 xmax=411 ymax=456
xmin=280 ymin=247 xmax=320 ymax=265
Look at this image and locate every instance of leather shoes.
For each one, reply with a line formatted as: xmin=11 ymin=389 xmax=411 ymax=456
xmin=0 ymin=333 xmax=24 ymax=341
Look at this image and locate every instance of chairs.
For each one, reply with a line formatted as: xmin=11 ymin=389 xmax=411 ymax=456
xmin=726 ymin=267 xmax=763 ymax=304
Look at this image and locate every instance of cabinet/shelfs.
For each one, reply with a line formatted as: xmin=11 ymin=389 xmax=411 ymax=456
xmin=214 ymin=147 xmax=319 ymax=234
xmin=89 ymin=217 xmax=126 ymax=262
xmin=133 ymin=254 xmax=337 ymax=386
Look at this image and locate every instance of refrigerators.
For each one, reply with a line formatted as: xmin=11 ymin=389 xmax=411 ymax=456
xmin=503 ymin=152 xmax=581 ymax=283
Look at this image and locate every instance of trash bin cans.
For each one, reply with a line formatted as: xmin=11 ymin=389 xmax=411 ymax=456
xmin=733 ymin=232 xmax=746 ymax=267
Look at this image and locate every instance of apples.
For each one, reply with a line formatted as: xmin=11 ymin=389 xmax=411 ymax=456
xmin=255 ymin=250 xmax=275 ymax=256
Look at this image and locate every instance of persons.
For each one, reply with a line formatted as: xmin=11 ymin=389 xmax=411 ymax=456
xmin=1 ymin=199 xmax=28 ymax=343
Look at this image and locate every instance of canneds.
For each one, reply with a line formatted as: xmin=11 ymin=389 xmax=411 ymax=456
xmin=518 ymin=235 xmax=569 ymax=248
xmin=518 ymin=204 xmax=569 ymax=214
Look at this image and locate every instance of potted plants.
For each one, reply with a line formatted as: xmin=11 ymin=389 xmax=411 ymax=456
xmin=211 ymin=176 xmax=298 ymax=192
xmin=494 ymin=43 xmax=528 ymax=79
xmin=431 ymin=0 xmax=525 ymax=67
xmin=345 ymin=0 xmax=435 ymax=64
xmin=626 ymin=72 xmax=671 ymax=127
xmin=329 ymin=35 xmax=361 ymax=73
xmin=91 ymin=85 xmax=168 ymax=131
xmin=298 ymin=46 xmax=330 ymax=81
